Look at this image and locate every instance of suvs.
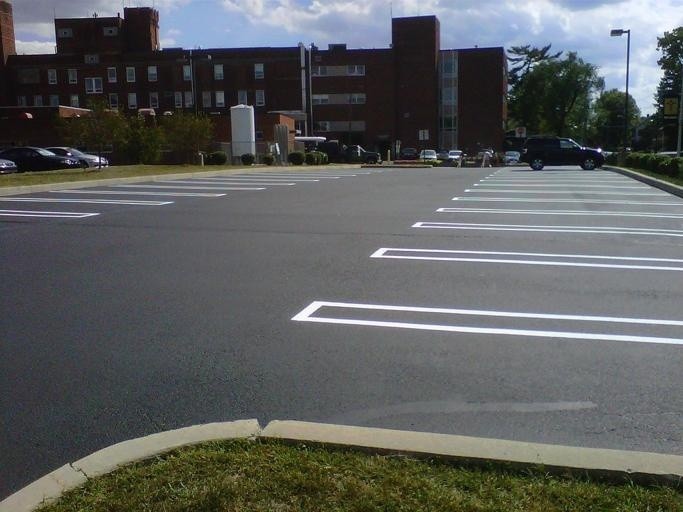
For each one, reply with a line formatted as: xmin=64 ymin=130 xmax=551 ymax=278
xmin=519 ymin=133 xmax=604 ymax=173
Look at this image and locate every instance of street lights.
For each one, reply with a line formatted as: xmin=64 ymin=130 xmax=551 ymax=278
xmin=187 ymin=50 xmax=213 ymax=167
xmin=609 ymin=27 xmax=630 ymax=152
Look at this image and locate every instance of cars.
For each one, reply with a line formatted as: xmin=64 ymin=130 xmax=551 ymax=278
xmin=399 ymin=147 xmax=523 ymax=165
xmin=1 ymin=146 xmax=109 ymax=174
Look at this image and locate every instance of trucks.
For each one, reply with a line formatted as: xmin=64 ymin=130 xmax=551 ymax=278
xmin=316 ymin=140 xmax=382 ymax=162
